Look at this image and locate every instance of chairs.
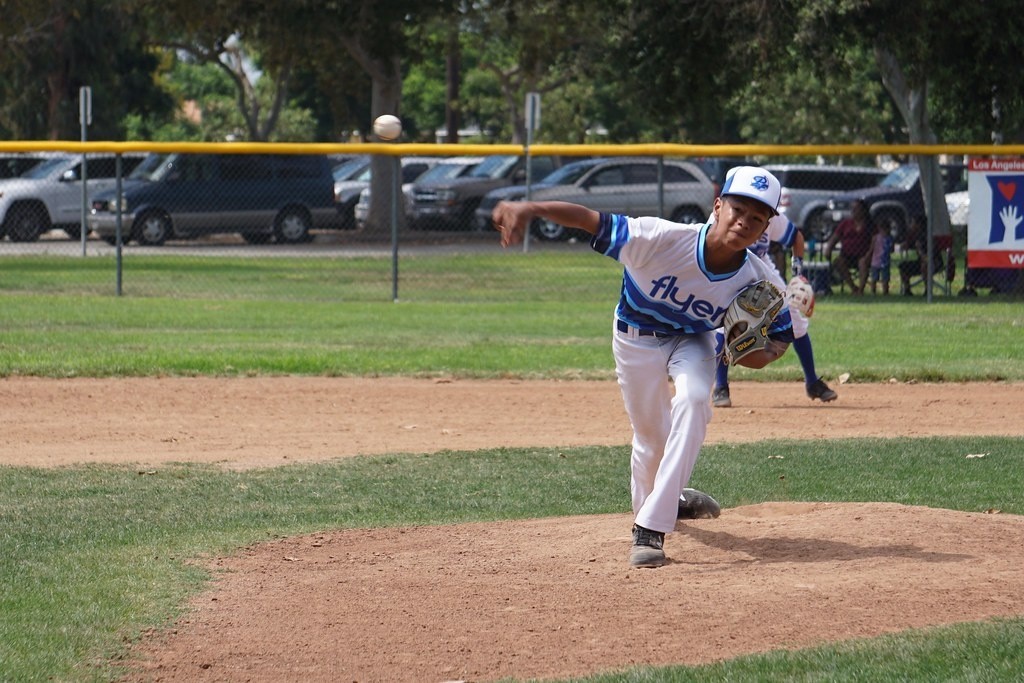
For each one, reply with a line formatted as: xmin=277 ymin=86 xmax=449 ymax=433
xmin=901 ymin=235 xmax=953 ymax=297
xmin=170 ymin=161 xmax=188 ymax=182
xmin=828 ymin=248 xmax=882 ymax=295
xmin=202 ymin=161 xmax=226 ymax=181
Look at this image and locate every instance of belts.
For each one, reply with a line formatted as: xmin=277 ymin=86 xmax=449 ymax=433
xmin=617 ymin=319 xmax=668 ymax=338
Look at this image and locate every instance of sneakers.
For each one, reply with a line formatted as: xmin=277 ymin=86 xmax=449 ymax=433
xmin=805 ymin=375 xmax=837 ymax=402
xmin=630 ymin=522 xmax=666 ymax=568
xmin=711 ymin=386 xmax=731 ymax=407
xmin=677 ymin=488 xmax=720 ymax=520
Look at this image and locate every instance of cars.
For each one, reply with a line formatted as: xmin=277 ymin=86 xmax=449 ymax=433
xmin=476 ymin=156 xmax=720 ymax=242
xmin=333 ymin=155 xmax=597 ymax=228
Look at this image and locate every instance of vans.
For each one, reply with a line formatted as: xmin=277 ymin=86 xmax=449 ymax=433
xmin=88 ymin=151 xmax=336 ymax=245
xmin=759 ymin=165 xmax=888 ymax=242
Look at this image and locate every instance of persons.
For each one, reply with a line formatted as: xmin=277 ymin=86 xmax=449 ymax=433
xmin=707 ymin=194 xmax=839 ymax=408
xmin=898 ymin=212 xmax=945 ymax=298
xmin=823 ymin=198 xmax=879 ymax=296
xmin=491 ymin=165 xmax=817 ymax=569
xmin=859 ymin=222 xmax=894 ymax=297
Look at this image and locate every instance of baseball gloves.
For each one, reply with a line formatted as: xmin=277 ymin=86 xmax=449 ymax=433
xmin=786 ymin=275 xmax=816 ymax=318
xmin=722 ymin=280 xmax=784 ymax=367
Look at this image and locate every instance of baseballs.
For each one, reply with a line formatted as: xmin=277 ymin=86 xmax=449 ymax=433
xmin=373 ymin=114 xmax=402 ymax=141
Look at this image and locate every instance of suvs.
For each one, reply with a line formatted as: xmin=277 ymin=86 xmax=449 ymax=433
xmin=0 ymin=158 xmax=146 ymax=243
xmin=822 ymin=161 xmax=969 ymax=244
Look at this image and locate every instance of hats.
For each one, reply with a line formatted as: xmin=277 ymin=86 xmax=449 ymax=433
xmin=721 ymin=166 xmax=781 ymax=216
